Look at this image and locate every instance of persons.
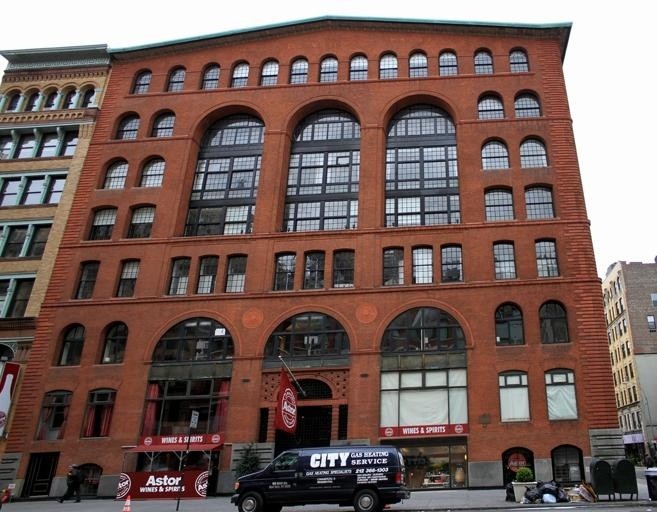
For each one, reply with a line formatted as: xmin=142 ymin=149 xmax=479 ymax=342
xmin=64 ymin=465 xmax=73 ymax=487
xmin=55 ymin=463 xmax=81 ymax=504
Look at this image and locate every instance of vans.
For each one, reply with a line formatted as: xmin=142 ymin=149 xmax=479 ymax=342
xmin=231 ymin=445 xmax=410 ymax=512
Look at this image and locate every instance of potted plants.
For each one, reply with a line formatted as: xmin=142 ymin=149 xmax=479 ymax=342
xmin=512 ymin=468 xmax=538 ymax=503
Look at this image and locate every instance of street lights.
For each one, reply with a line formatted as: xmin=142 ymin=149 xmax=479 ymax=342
xmin=623 ymin=382 xmax=655 ymax=440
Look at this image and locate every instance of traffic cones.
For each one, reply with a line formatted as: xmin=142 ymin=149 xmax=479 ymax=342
xmin=121 ymin=495 xmax=131 ymax=512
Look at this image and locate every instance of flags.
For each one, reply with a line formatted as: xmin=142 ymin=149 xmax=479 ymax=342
xmin=273 ymin=366 xmax=298 ymax=434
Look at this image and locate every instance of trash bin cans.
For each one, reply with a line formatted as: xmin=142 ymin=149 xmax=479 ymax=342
xmin=644 ymin=470 xmax=657 ymax=501
xmin=590 ymin=459 xmax=638 ymax=500
